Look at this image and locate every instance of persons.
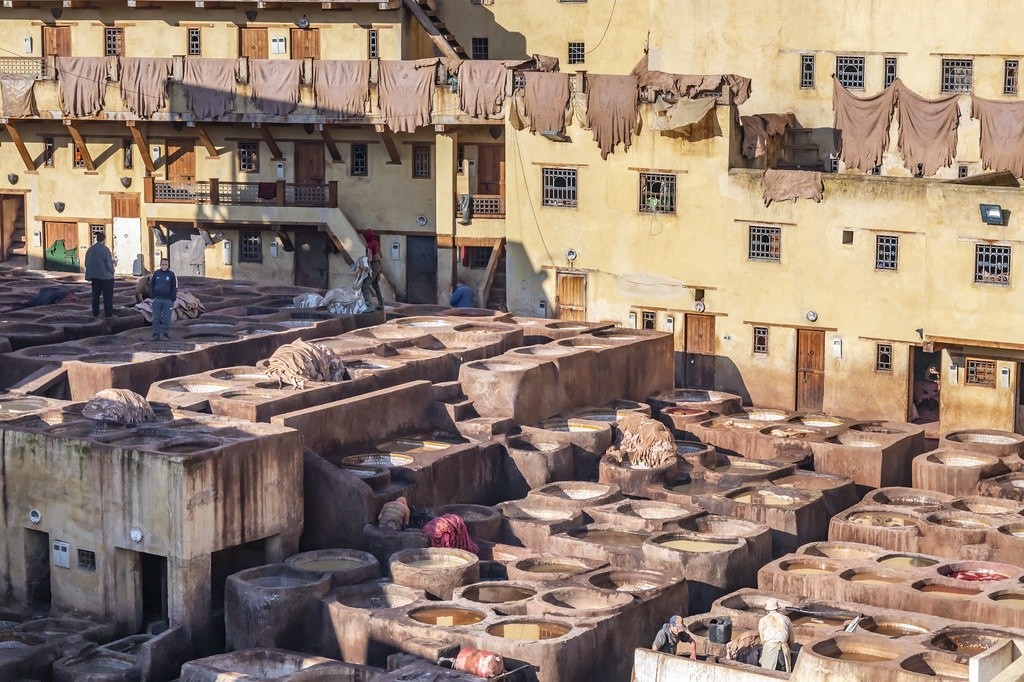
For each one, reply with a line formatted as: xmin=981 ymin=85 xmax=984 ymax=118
xmin=134 ymin=274 xmax=153 ymax=304
xmin=450 ymin=279 xmax=475 ymax=308
xmin=652 ymin=616 xmax=697 ymax=660
xmin=758 ymin=599 xmax=794 ymax=673
xmin=378 ymin=496 xmax=411 ymax=532
xmin=84 ymin=234 xmax=119 ymax=319
xmin=360 ymin=228 xmax=384 ymax=314
xmin=150 ymin=258 xmax=178 ymax=341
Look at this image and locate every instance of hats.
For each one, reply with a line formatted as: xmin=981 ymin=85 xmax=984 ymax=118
xmin=765 ymin=599 xmax=781 ymax=610
xmin=670 ymin=615 xmax=688 ymax=631
